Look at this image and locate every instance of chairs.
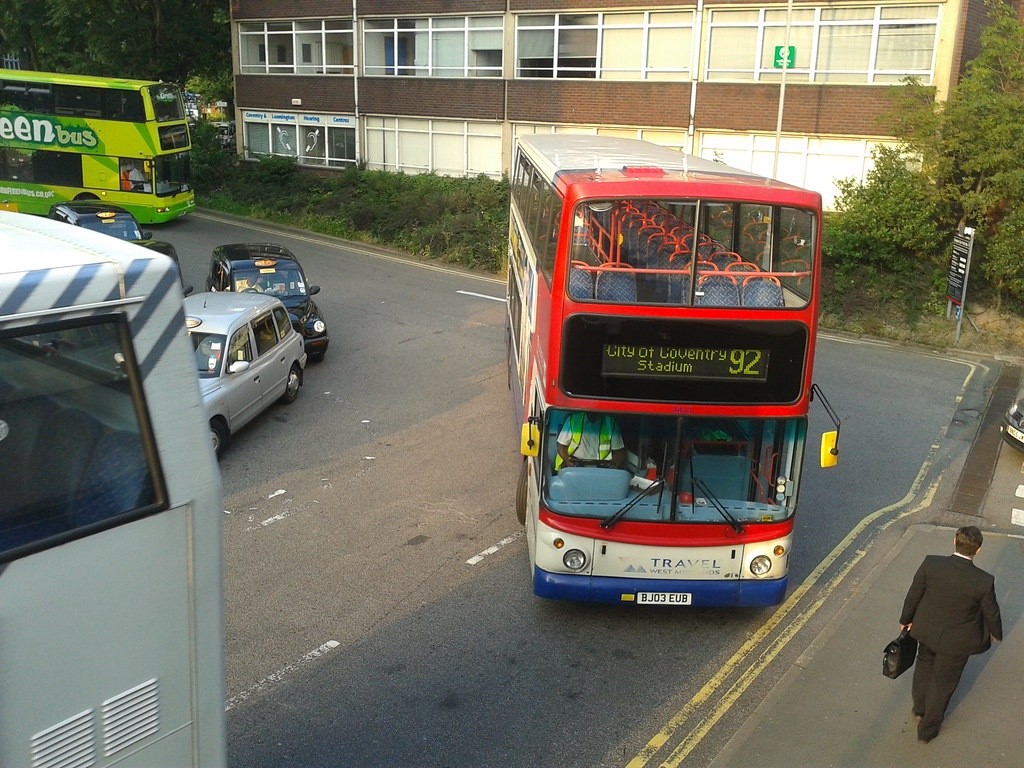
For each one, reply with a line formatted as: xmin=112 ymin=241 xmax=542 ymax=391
xmin=535 ymin=186 xmax=785 ymax=308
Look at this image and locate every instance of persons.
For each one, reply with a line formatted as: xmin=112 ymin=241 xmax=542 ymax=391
xmin=223 ymin=270 xmax=264 ymax=292
xmin=900 ymin=525 xmax=1002 ymax=743
xmin=554 ymin=412 xmax=625 ymax=476
xmin=129 ymin=161 xmax=149 ymax=190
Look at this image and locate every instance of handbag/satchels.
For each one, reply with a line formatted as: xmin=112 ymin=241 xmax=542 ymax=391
xmin=882 ymin=626 xmax=917 ymax=680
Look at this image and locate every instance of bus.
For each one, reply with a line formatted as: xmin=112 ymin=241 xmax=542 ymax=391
xmin=504 ymin=134 xmax=843 ymax=610
xmin=1 ymin=67 xmax=199 ymax=225
xmin=0 ymin=209 xmax=228 ymax=768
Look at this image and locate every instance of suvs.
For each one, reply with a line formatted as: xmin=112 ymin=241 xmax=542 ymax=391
xmin=182 ymin=290 xmax=308 ymax=464
xmin=206 ymin=243 xmax=331 ymax=363
xmin=46 ymin=201 xmax=154 ymax=242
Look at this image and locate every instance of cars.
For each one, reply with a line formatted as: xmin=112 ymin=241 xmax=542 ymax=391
xmin=999 ymin=397 xmax=1024 ymax=452
xmin=158 ymin=85 xmax=236 ymax=153
